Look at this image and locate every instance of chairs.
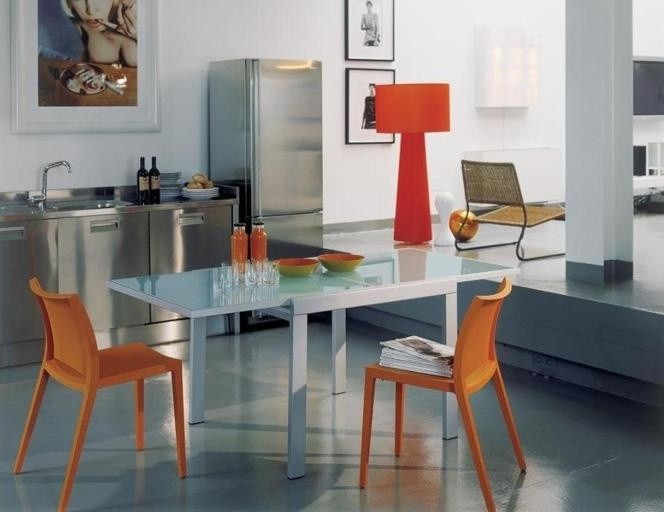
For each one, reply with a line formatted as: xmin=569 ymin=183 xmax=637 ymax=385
xmin=13 ymin=276 xmax=188 ymax=511
xmin=453 ymin=160 xmax=565 ymax=262
xmin=358 ymin=277 xmax=527 ymax=511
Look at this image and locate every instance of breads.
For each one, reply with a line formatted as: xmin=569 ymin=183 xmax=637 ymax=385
xmin=186 ymin=173 xmax=212 ymax=189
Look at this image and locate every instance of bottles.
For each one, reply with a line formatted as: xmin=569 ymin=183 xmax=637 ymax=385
xmin=230 ymin=223 xmax=248 ymax=272
xmin=250 ymin=221 xmax=267 ymax=259
xmin=136 ymin=156 xmax=161 ymax=205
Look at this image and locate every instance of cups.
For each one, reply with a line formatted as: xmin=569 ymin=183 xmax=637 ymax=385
xmin=210 ymin=259 xmax=279 ymax=290
xmin=212 ymin=286 xmax=278 ymax=308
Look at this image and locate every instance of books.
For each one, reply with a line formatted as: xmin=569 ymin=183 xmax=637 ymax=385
xmin=378 ymin=335 xmax=455 ymax=378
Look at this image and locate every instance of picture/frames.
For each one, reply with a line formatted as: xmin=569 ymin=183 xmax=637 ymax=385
xmin=344 ymin=66 xmax=396 ymax=144
xmin=9 ymin=0 xmax=160 ymax=135
xmin=344 ymin=2 xmax=395 ymax=61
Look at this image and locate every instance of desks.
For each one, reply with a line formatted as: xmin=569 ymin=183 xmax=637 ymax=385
xmin=104 ymin=250 xmax=523 ymax=480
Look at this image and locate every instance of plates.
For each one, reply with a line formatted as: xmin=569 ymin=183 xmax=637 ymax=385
xmin=159 ymin=170 xmax=219 ymax=200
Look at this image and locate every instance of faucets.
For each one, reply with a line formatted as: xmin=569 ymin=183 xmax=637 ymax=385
xmin=42 ymin=160 xmax=73 ymax=196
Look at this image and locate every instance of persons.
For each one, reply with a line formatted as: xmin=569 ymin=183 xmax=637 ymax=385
xmin=361 ymin=84 xmax=376 ymax=130
xmin=60 ymin=0 xmax=137 ymax=66
xmin=360 ymin=1 xmax=381 ymax=45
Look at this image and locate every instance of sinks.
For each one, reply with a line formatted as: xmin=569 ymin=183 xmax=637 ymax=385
xmin=45 ymin=198 xmax=134 ymax=213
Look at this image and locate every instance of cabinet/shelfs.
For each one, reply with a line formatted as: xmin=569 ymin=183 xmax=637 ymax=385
xmin=58 ymin=209 xmax=149 ymax=352
xmin=0 ymin=216 xmax=58 ymax=367
xmin=148 ymin=191 xmax=240 ymax=348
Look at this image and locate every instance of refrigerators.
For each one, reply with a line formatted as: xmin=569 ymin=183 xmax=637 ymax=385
xmin=207 ymin=59 xmax=323 ymax=261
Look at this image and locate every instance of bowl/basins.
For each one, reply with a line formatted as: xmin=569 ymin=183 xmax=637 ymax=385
xmin=317 ymin=253 xmax=365 ymax=271
xmin=272 ymin=258 xmax=321 ymax=277
xmin=279 ymin=270 xmax=365 ymax=294
